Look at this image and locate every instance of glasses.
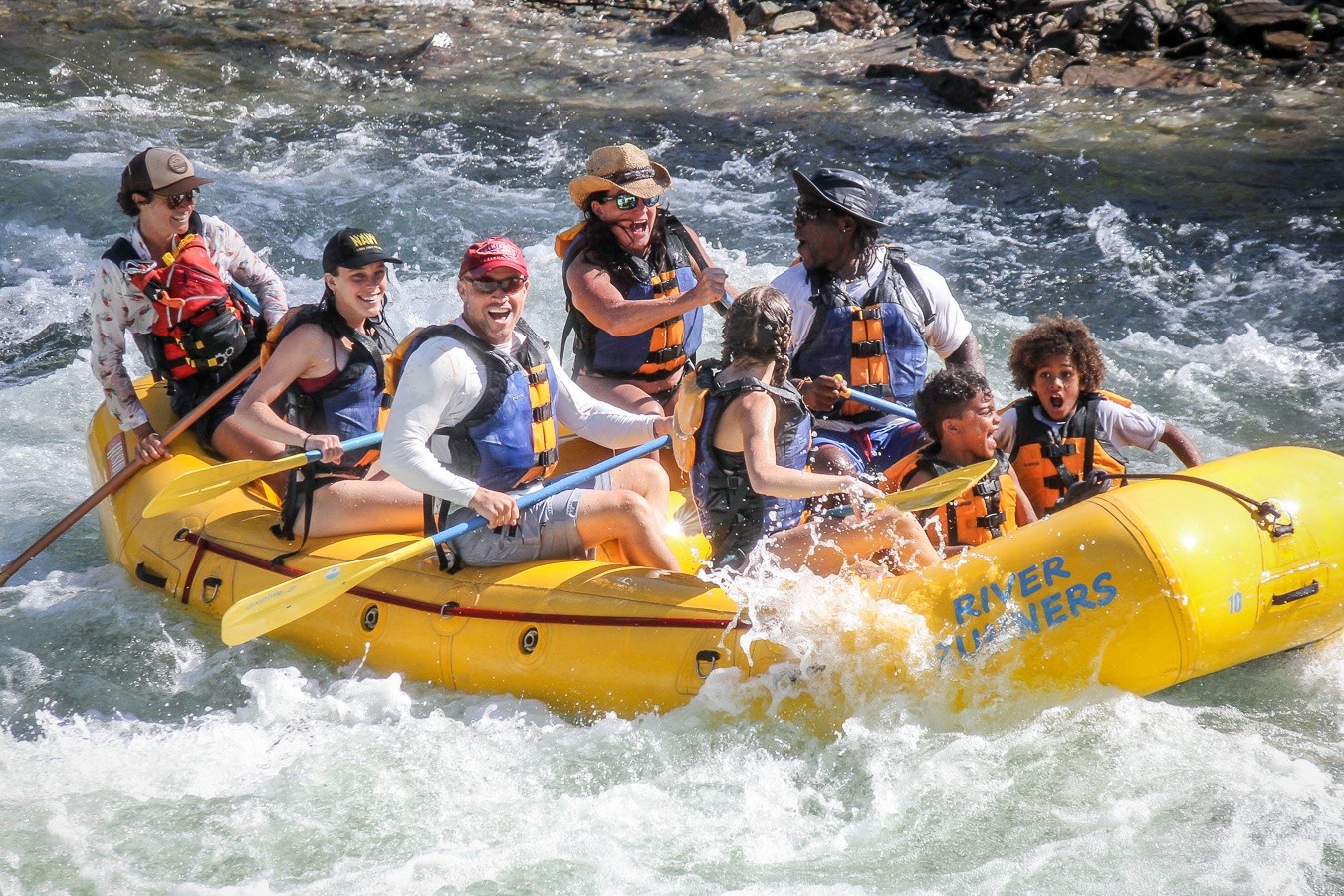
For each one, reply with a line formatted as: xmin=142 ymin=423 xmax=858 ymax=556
xmin=460 ymin=274 xmax=528 ymax=293
xmin=794 ymin=203 xmax=844 ymax=222
xmin=598 ymin=193 xmax=659 ymax=211
xmin=144 ymin=187 xmax=201 ymax=209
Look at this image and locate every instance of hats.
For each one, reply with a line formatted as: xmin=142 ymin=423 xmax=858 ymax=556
xmin=322 ymin=226 xmax=403 ymax=272
xmin=569 ymin=143 xmax=672 ymax=211
xmin=130 ymin=147 xmax=214 ymax=197
xmin=460 ymin=237 xmax=528 ymax=278
xmin=791 ymin=165 xmax=891 ymax=229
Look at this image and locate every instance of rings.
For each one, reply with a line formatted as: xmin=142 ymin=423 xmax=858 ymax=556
xmin=302 ymin=435 xmax=313 ymax=450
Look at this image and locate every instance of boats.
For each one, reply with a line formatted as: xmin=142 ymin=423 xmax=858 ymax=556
xmin=83 ymin=368 xmax=1344 ymax=751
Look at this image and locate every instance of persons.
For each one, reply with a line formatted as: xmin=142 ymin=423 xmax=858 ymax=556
xmin=91 ymin=146 xmax=299 ymax=479
xmin=233 ymin=225 xmax=438 ymax=538
xmin=698 ymin=286 xmax=944 ymax=590
xmin=380 ymin=236 xmax=686 ymax=572
xmin=765 ymin=163 xmax=983 ymax=506
xmin=989 ymin=323 xmax=1204 ymax=510
xmin=563 ymin=143 xmax=730 ymax=462
xmin=901 ymin=368 xmax=1039 ymax=555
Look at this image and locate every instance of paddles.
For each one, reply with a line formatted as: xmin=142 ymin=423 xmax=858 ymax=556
xmin=809 ymin=457 xmax=998 ymax=523
xmin=220 ymin=435 xmax=673 ymax=647
xmin=0 ymin=357 xmax=263 ymax=587
xmin=719 ymin=289 xmax=734 ymax=309
xmin=141 ymin=431 xmax=386 ymax=518
xmin=832 ymin=374 xmax=920 ymax=423
